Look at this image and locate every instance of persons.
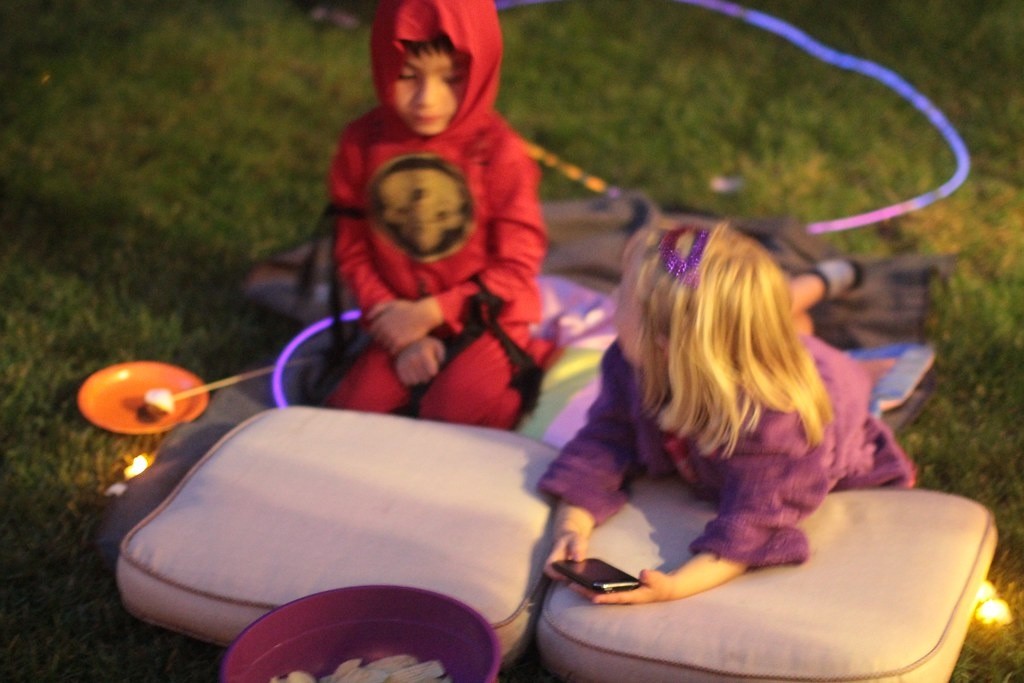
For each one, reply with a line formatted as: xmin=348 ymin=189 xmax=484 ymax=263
xmin=313 ymin=0 xmax=559 ymax=432
xmin=537 ymin=211 xmax=919 ymax=605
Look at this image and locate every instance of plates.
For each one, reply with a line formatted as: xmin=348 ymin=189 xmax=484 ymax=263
xmin=75 ymin=361 xmax=211 ymax=437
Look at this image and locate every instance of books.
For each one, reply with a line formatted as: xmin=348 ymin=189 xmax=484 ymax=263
xmin=837 ymin=345 xmax=935 ymax=412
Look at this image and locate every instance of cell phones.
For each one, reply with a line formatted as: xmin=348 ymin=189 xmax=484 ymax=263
xmin=551 ymin=559 xmax=640 ymax=595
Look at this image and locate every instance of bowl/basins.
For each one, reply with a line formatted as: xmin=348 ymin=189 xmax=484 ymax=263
xmin=218 ymin=583 xmax=503 ymax=683
